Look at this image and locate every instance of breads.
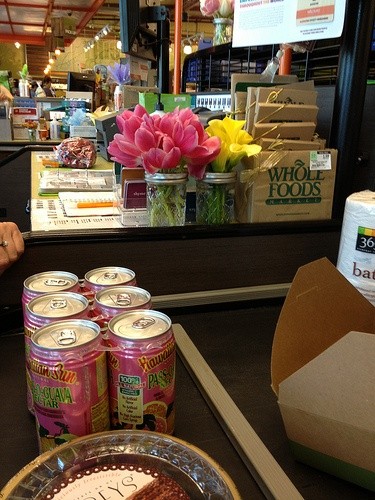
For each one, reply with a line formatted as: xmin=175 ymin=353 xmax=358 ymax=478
xmin=54 ymin=137 xmax=97 ymax=169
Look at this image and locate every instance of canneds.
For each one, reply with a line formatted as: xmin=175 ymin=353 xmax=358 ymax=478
xmin=93 ymin=286 xmax=153 ymax=333
xmin=110 ymin=309 xmax=176 ymax=436
xmin=23 ymin=270 xmax=79 ymax=317
xmin=84 ymin=267 xmax=135 ymax=303
xmin=30 ymin=319 xmax=108 ymax=456
xmin=25 ymin=292 xmax=89 ymax=416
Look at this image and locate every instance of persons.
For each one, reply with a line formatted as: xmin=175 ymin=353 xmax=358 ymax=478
xmin=0 ymin=70 xmax=56 ymax=101
xmin=0 ymin=221 xmax=24 ymax=275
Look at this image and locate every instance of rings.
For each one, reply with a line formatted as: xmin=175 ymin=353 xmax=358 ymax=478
xmin=0 ymin=241 xmax=8 ymax=246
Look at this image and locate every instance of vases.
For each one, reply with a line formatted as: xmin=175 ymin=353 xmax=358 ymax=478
xmin=146 ymin=170 xmax=187 ymax=227
xmin=196 ymin=170 xmax=237 ymax=225
xmin=212 ymin=17 xmax=233 ymax=46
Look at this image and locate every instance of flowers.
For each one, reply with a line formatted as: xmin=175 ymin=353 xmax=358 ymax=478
xmin=108 ymin=104 xmax=221 ymax=222
xmin=197 ymin=105 xmax=263 ymax=221
xmin=200 ymin=0 xmax=235 ymax=43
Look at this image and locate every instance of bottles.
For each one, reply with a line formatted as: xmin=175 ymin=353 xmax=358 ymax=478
xmin=49 ymin=116 xmax=61 ymax=139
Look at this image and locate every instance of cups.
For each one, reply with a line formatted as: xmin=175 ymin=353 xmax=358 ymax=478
xmin=39 ymin=129 xmax=47 ymax=141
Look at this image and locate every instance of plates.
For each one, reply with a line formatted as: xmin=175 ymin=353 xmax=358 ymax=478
xmin=0 ymin=430 xmax=242 ymax=500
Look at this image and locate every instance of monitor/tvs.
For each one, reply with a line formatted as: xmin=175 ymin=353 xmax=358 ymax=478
xmin=120 ymin=0 xmax=158 ymax=62
xmin=68 ymin=72 xmax=95 ymax=93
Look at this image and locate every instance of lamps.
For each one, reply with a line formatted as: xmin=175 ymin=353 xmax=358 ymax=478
xmin=84 ymin=24 xmax=111 ymax=51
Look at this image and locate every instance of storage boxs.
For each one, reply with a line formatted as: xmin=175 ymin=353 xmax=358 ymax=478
xmin=120 ymin=87 xmax=192 ymax=113
xmin=271 ymin=257 xmax=374 ymax=492
